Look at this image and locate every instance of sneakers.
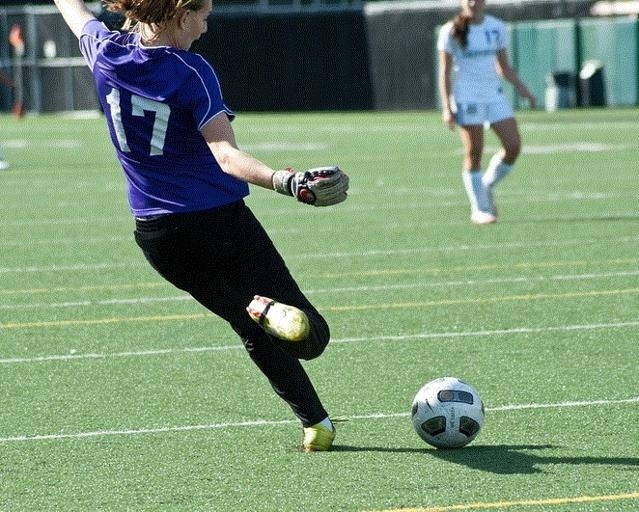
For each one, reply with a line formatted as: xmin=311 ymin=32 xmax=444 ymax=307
xmin=244 ymin=293 xmax=312 ymax=343
xmin=470 ymin=191 xmax=499 ymax=225
xmin=301 ymin=419 xmax=338 ymax=452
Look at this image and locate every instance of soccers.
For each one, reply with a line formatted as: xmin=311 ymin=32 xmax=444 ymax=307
xmin=411 ymin=377 xmax=485 ymax=448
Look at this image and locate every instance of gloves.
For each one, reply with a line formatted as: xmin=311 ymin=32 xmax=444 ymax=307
xmin=270 ymin=162 xmax=352 ymax=210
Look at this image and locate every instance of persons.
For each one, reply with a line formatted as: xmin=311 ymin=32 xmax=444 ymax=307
xmin=435 ymin=0 xmax=539 ymax=228
xmin=50 ymin=0 xmax=350 ymax=454
